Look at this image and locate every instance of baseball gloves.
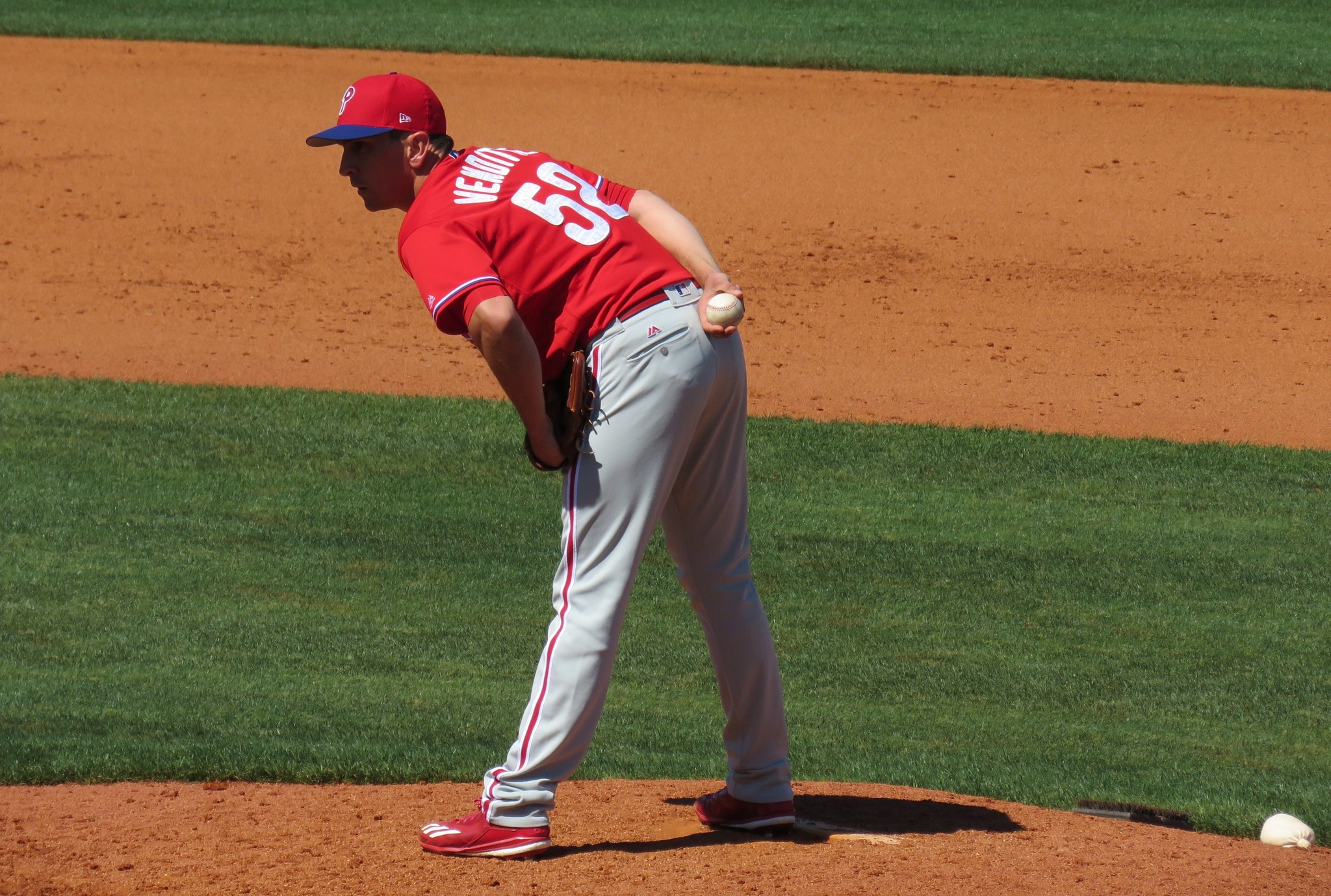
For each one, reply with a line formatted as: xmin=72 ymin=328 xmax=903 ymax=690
xmin=525 ymin=349 xmax=597 ymax=473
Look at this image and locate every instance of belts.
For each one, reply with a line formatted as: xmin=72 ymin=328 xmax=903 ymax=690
xmin=618 ymin=280 xmax=699 ymax=319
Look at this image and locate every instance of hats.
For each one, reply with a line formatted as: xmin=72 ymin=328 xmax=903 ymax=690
xmin=306 ymin=72 xmax=446 ymax=148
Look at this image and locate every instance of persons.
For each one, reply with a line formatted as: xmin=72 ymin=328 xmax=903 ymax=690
xmin=307 ymin=73 xmax=797 ymax=860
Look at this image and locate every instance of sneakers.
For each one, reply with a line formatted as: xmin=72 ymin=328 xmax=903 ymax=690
xmin=692 ymin=786 xmax=796 ymax=830
xmin=419 ymin=798 xmax=551 ymax=860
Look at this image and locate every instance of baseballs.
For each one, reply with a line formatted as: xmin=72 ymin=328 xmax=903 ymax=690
xmin=706 ymin=293 xmax=745 ymax=328
